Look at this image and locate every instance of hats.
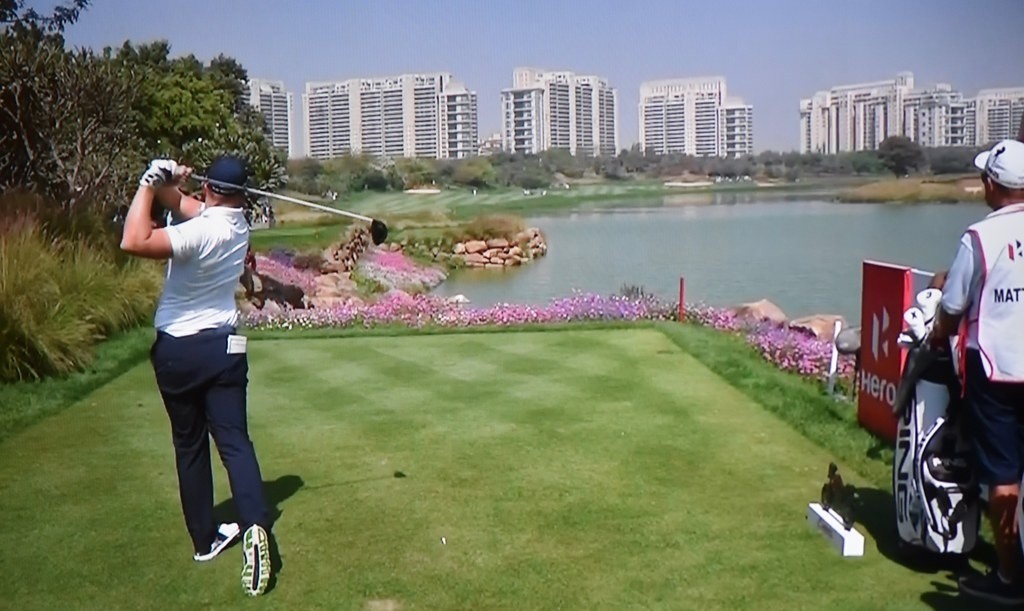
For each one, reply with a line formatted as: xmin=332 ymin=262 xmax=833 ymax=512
xmin=975 ymin=139 xmax=1024 ymax=189
xmin=205 ymin=155 xmax=251 ymax=194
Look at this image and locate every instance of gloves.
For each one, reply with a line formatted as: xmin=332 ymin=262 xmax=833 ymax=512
xmin=138 ymin=157 xmax=178 ymax=186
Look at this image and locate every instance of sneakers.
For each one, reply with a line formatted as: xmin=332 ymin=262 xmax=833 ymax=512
xmin=239 ymin=524 xmax=272 ymax=596
xmin=958 ymin=565 xmax=1024 ymax=604
xmin=190 ymin=522 xmax=241 ymax=562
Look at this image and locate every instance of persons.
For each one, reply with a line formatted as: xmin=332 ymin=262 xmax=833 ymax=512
xmin=929 ymin=140 xmax=1023 ymax=606
xmin=119 ymin=157 xmax=272 ymax=598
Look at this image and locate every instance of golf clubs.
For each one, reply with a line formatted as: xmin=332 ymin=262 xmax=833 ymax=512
xmin=146 ymin=162 xmax=389 ymax=246
